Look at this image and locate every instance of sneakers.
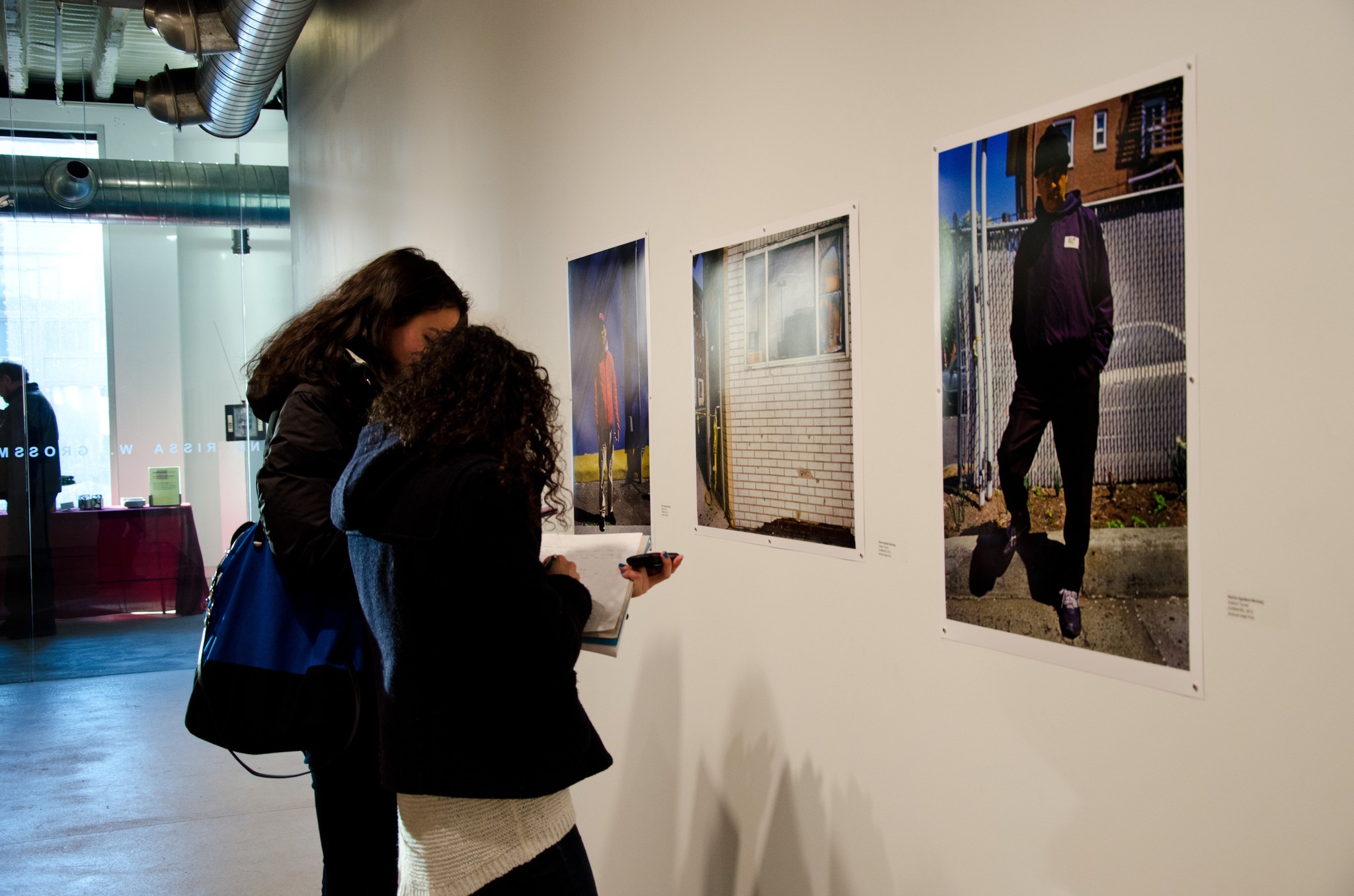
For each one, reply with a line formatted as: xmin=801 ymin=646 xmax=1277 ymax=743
xmin=1058 ymin=586 xmax=1082 ymax=640
xmin=996 ymin=514 xmax=1031 ymax=555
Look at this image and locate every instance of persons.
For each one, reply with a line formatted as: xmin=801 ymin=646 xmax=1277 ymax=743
xmin=997 ymin=122 xmax=1115 ymax=637
xmin=240 ymin=247 xmax=467 ymax=896
xmin=0 ymin=360 xmax=62 ymax=638
xmin=328 ymin=326 xmax=686 ymax=896
xmin=592 ymin=312 xmax=620 ymax=528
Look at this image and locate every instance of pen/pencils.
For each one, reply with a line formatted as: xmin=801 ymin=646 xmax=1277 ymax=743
xmin=544 ymin=553 xmax=558 ymax=570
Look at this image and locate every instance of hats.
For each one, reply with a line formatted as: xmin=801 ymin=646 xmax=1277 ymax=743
xmin=1033 ymin=124 xmax=1071 ymax=177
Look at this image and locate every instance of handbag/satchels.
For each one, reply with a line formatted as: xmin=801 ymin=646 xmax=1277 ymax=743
xmin=185 ymin=519 xmax=359 ymax=752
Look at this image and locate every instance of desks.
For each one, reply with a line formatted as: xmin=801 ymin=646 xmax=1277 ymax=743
xmin=0 ymin=502 xmax=209 ymax=625
